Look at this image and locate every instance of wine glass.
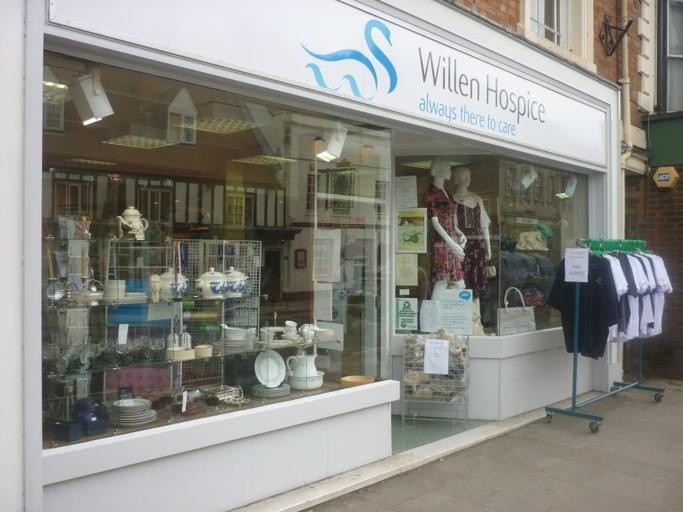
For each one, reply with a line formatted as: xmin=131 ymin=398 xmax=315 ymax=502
xmin=47 ymin=334 xmax=166 ymax=373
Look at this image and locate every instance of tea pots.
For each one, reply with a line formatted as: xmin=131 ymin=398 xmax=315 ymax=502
xmin=223 ymin=265 xmax=247 ymax=299
xmin=116 ymin=207 xmax=148 ymax=243
xmin=285 ymin=354 xmax=317 ymax=376
xmin=159 ymin=267 xmax=189 ymax=304
xmin=297 ymin=324 xmax=315 ymax=342
xmin=194 ymin=266 xmax=226 ymax=300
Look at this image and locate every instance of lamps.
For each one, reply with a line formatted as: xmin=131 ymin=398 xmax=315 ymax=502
xmin=316 ymin=122 xmax=348 ymax=163
xmin=68 ymin=74 xmax=114 ymax=126
xmin=554 ymin=173 xmax=578 ymax=199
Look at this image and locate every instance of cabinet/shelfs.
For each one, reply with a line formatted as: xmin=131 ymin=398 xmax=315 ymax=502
xmin=42 ymin=235 xmax=341 ymax=450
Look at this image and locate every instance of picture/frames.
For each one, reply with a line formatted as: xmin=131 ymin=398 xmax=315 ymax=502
xmin=295 ymin=248 xmax=307 ymax=268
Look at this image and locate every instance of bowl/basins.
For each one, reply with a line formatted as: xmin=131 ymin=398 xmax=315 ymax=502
xmin=224 ymin=327 xmax=247 ymax=340
xmin=340 ymin=376 xmax=374 ymax=388
xmin=286 ymin=371 xmax=325 ymax=389
xmin=259 ymin=320 xmax=296 ymax=341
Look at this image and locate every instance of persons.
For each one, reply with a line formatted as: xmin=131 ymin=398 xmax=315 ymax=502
xmin=454 ymin=165 xmax=492 ymax=324
xmin=145 ymin=274 xmax=166 ymax=302
xmin=423 ymin=156 xmax=467 ymax=302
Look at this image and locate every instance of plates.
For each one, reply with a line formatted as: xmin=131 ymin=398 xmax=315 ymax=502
xmin=108 ymin=399 xmax=157 ymax=425
xmin=250 ymin=382 xmax=289 ymax=397
xmin=253 ymin=350 xmax=286 ymax=388
xmin=212 ymin=340 xmax=251 ymax=354
xmin=102 ymin=278 xmax=147 ymax=304
xmin=164 ymin=344 xmax=211 ymax=361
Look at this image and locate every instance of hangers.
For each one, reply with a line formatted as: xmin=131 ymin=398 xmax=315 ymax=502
xmin=585 ymin=239 xmax=642 ymax=256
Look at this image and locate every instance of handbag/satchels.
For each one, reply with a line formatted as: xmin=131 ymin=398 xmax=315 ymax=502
xmin=497 ymin=287 xmax=536 ymax=335
xmin=516 ymin=231 xmax=549 ymax=251
xmin=486 ymin=266 xmax=496 ymax=277
